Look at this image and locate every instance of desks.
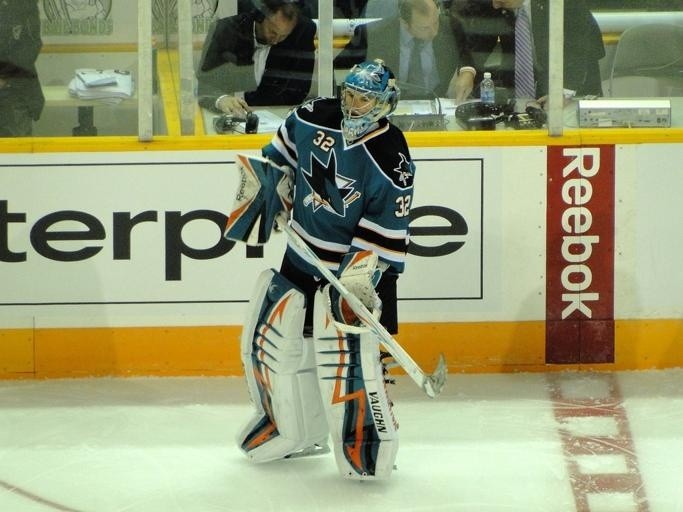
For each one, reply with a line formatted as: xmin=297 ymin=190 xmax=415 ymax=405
xmin=40 ymin=83 xmax=139 ymax=136
xmin=203 ymin=98 xmax=682 ymax=134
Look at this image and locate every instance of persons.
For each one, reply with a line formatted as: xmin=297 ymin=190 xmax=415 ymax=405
xmin=333 ymin=0 xmax=501 ymax=104
xmin=220 ymin=57 xmax=417 ymax=483
xmin=493 ymin=0 xmax=607 ymax=102
xmin=0 ymin=1 xmax=47 ymax=137
xmin=198 ymin=0 xmax=318 ymax=118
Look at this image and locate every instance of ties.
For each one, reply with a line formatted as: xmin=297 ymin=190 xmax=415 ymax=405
xmin=513 ymin=5 xmax=537 ymax=103
xmin=406 ymin=41 xmax=428 ymax=104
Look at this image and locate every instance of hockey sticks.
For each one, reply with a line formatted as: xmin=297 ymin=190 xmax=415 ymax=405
xmin=275 ymin=216 xmax=447 ymax=398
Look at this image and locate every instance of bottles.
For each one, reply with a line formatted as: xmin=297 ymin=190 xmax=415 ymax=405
xmin=479 ymin=72 xmax=495 ymax=103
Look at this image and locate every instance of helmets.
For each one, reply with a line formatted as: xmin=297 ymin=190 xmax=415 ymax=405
xmin=341 ymin=60 xmax=402 ymax=141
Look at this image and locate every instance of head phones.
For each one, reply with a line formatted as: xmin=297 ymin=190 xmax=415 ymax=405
xmin=526 ymin=104 xmax=548 ymax=125
xmin=215 ymin=111 xmax=259 ymax=133
xmin=250 ymin=9 xmax=265 ymax=22
xmin=456 ymin=102 xmax=512 ymax=126
xmin=399 ymin=2 xmax=448 ymax=23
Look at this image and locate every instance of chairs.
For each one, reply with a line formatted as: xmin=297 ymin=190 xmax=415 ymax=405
xmin=611 ymin=22 xmax=683 ymax=99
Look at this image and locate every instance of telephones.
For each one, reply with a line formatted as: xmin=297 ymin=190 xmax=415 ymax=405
xmin=504 ymin=106 xmax=547 ymax=130
